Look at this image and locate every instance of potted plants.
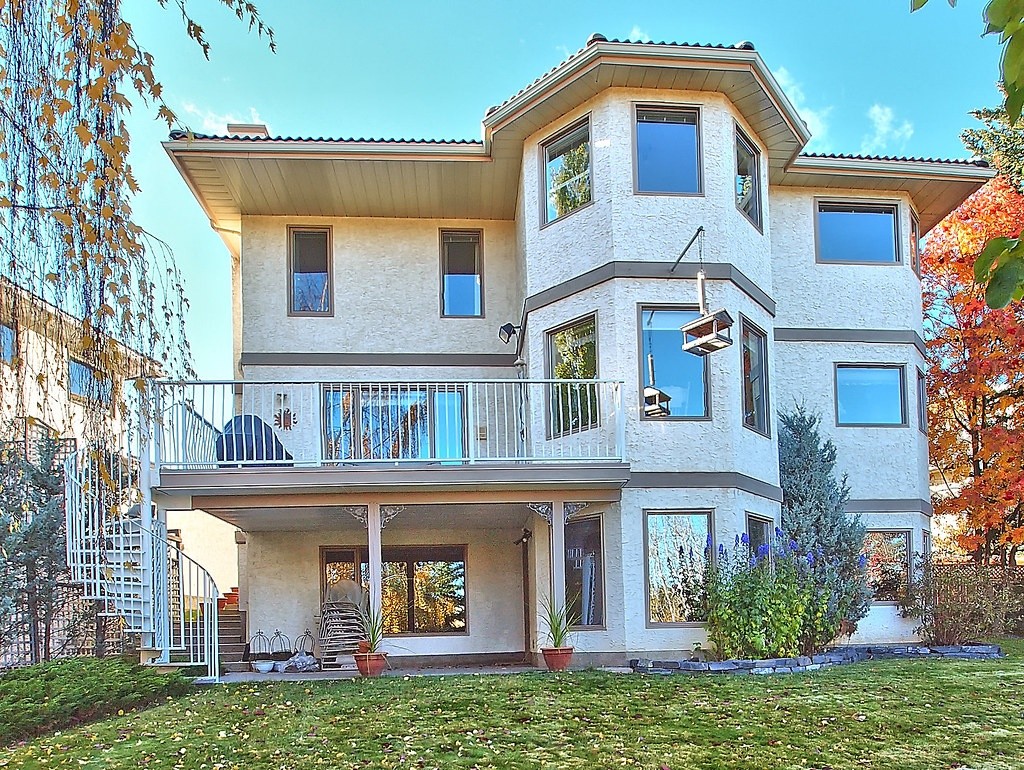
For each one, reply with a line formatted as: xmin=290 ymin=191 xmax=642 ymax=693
xmin=526 ymin=587 xmax=584 ymax=672
xmin=352 ymin=602 xmax=388 ymax=677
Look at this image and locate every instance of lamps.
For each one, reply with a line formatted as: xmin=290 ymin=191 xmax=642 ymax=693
xmin=499 ymin=323 xmax=521 ymax=345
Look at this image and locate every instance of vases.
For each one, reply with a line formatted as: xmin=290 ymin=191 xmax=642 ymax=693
xmin=223 ymin=592 xmax=239 ymax=604
xmin=255 ymin=661 xmax=275 ymax=674
xmin=210 ymin=597 xmax=228 ymax=611
xmin=200 ymin=603 xmax=214 ymax=615
xmin=230 ymin=587 xmax=239 ymax=593
